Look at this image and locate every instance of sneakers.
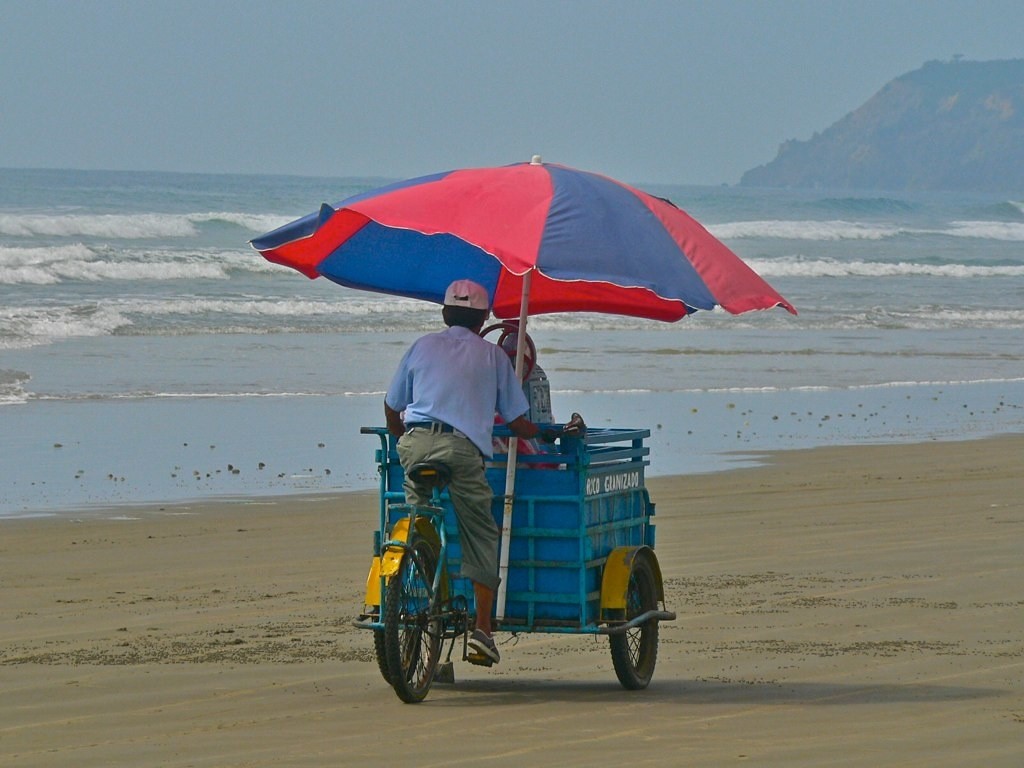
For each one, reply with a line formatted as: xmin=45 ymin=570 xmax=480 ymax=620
xmin=467 ymin=628 xmax=501 ymax=665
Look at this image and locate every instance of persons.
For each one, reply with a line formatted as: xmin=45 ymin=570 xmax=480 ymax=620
xmin=383 ymin=276 xmax=539 ymax=663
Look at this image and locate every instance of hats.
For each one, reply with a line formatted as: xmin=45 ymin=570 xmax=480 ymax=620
xmin=443 ymin=278 xmax=490 ymax=310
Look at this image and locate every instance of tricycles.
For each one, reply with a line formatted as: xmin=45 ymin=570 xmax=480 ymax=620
xmin=350 ymin=421 xmax=677 ymax=708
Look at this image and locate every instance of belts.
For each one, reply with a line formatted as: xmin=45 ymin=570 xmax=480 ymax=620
xmin=406 ymin=421 xmax=455 ymax=434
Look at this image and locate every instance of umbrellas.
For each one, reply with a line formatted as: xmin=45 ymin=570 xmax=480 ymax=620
xmin=242 ymin=153 xmax=802 ymax=622
